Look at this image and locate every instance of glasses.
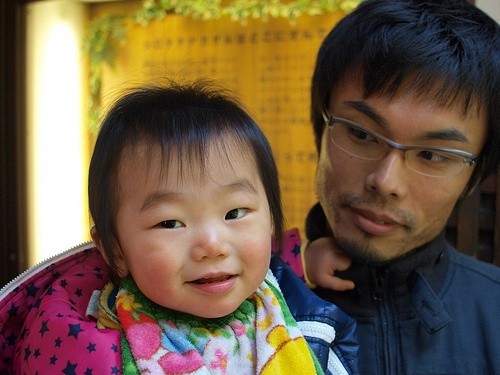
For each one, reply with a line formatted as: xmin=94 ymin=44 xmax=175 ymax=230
xmin=321 ymin=108 xmax=487 ymax=178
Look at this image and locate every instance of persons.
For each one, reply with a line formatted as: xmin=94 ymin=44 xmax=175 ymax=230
xmin=0 ymin=76 xmax=359 ymax=375
xmin=294 ymin=0 xmax=500 ymax=375
